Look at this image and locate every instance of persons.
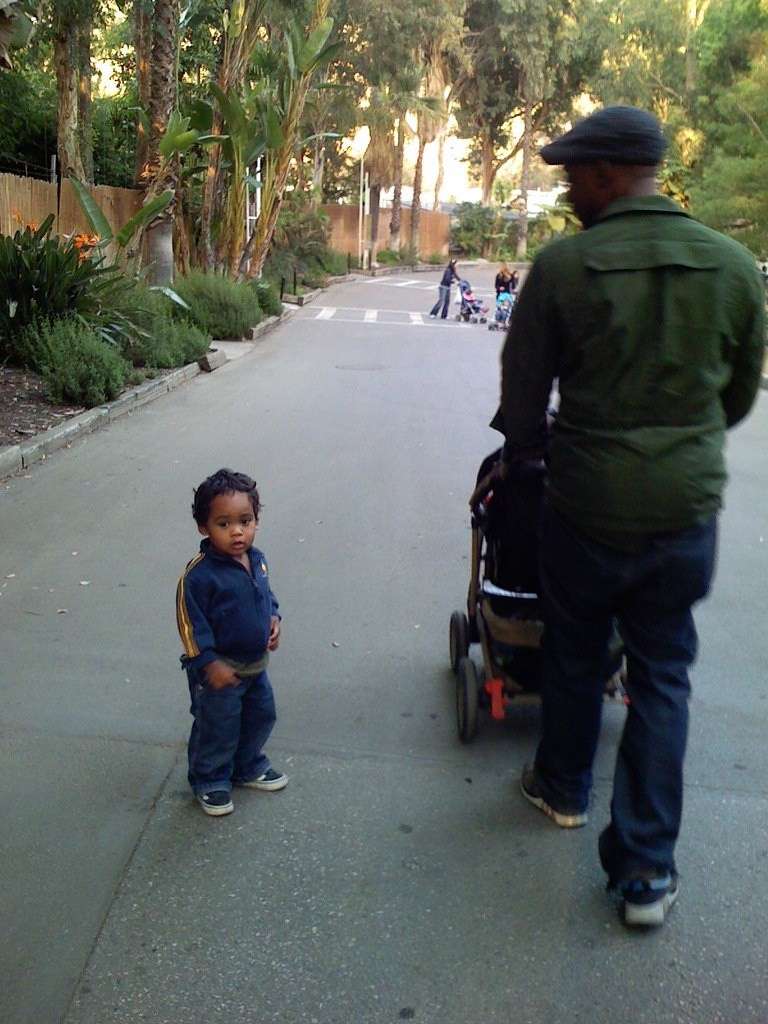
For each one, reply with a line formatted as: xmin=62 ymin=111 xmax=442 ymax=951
xmin=485 ymin=105 xmax=765 ymax=924
xmin=430 ymin=256 xmax=462 ymax=319
xmin=466 ymin=264 xmax=519 ymax=321
xmin=176 ymin=467 xmax=289 ymax=815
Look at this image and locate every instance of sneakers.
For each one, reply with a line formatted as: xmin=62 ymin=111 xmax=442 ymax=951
xmin=196 ymin=791 xmax=234 ymax=816
xmin=232 ymin=767 xmax=289 ymax=791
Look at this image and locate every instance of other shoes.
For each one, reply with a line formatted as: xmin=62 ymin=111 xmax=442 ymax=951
xmin=521 ymin=762 xmax=588 ymax=826
xmin=442 ymin=316 xmax=449 ymax=319
xmin=430 ymin=314 xmax=435 ymax=319
xmin=480 ymin=306 xmax=489 ymax=313
xmin=624 ymin=872 xmax=678 ymax=925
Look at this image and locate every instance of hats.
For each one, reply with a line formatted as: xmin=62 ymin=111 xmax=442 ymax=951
xmin=540 ymin=106 xmax=662 ymax=165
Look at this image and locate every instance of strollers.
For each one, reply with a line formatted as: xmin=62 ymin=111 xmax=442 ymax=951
xmin=446 ymin=407 xmax=632 ymax=745
xmin=487 ymin=289 xmax=516 ymax=332
xmin=454 ymin=281 xmax=490 ymax=324
